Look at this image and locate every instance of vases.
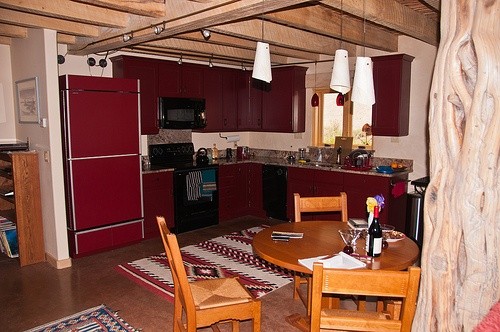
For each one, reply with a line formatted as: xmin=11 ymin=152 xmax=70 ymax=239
xmin=368 ymin=213 xmax=374 ymax=228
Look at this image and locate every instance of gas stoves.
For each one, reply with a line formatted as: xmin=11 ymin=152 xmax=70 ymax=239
xmin=149 ymin=142 xmax=219 ymax=174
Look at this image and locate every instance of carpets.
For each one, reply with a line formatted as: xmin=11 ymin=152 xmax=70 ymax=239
xmin=112 ymin=223 xmax=304 ymax=307
xmin=23 ymin=303 xmax=143 ymax=332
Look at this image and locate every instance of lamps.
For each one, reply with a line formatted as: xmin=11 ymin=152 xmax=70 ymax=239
xmin=311 ymin=0 xmax=376 ymax=107
xmin=124 ymin=21 xmax=245 ymax=71
xmin=252 ymin=0 xmax=273 ymax=83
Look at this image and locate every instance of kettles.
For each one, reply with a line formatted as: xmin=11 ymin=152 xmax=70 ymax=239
xmin=195 ymin=148 xmax=209 ymax=167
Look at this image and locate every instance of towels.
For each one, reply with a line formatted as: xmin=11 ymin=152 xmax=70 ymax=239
xmin=200 ymin=169 xmax=217 ymax=202
xmin=186 ymin=170 xmax=202 ymax=200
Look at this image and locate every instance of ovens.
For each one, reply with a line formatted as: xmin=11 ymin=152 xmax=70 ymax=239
xmin=173 ymin=169 xmax=220 ymax=234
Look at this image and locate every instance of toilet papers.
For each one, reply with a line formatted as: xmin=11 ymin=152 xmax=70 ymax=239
xmin=226 ymin=135 xmax=240 ymax=143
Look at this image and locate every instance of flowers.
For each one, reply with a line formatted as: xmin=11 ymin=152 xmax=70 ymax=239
xmin=366 ymin=194 xmax=385 ymax=213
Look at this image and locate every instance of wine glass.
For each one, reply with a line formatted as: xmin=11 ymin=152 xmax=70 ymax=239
xmin=338 ymin=229 xmax=362 ymax=255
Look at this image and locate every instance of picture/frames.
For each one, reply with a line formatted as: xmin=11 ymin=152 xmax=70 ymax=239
xmin=15 ymin=76 xmax=41 ymax=124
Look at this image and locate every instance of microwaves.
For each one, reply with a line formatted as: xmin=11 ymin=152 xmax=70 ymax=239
xmin=158 ymin=96 xmax=207 ymax=130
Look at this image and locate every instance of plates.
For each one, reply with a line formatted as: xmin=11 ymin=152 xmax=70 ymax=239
xmin=366 ymin=224 xmax=407 ymax=243
xmin=377 ymin=166 xmax=395 ymax=173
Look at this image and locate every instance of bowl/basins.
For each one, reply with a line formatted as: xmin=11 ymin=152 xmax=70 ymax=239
xmin=390 ymin=166 xmax=407 ymax=172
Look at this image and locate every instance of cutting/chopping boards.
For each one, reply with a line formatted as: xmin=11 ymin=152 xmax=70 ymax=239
xmin=333 ymin=136 xmax=353 ymax=164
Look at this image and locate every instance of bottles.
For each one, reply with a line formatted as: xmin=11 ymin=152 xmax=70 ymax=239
xmin=213 ymin=143 xmax=217 ymax=160
xmin=365 ymin=205 xmax=383 ymax=257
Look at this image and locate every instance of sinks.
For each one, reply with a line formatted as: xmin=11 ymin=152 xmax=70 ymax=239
xmin=304 ymin=163 xmax=336 ymax=167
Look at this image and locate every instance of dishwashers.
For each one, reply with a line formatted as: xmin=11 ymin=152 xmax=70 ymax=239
xmin=261 ymin=164 xmax=287 ymax=222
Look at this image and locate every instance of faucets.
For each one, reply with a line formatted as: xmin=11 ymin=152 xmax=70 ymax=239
xmin=336 ymin=145 xmax=342 ymax=165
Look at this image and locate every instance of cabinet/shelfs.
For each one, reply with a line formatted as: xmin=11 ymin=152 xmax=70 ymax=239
xmin=0 ymin=148 xmax=45 ymax=267
xmin=143 ymin=162 xmax=408 ymax=240
xmin=109 ymin=55 xmax=309 ymax=134
xmin=371 ymin=53 xmax=416 ymax=137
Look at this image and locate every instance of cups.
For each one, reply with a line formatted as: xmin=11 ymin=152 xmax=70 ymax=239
xmin=300 ymin=149 xmax=305 ymax=159
xmin=227 ymin=148 xmax=230 ymax=158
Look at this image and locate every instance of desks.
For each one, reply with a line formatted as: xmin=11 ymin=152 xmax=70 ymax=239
xmin=253 ymin=220 xmax=420 ymax=316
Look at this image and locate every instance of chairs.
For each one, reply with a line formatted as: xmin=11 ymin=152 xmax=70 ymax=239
xmin=156 ymin=216 xmax=262 ymax=332
xmin=310 ymin=261 xmax=422 ymax=332
xmin=293 ymin=192 xmax=366 ymax=316
